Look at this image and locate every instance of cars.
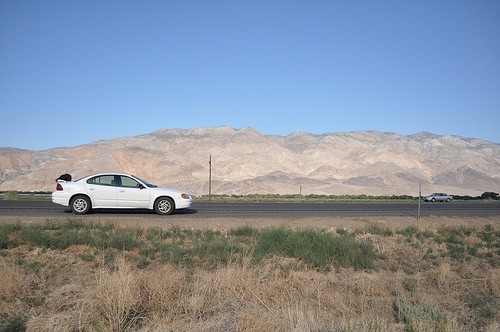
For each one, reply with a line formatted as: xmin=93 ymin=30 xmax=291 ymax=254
xmin=51 ymin=173 xmax=194 ymax=215
xmin=422 ymin=192 xmax=453 ymax=203
